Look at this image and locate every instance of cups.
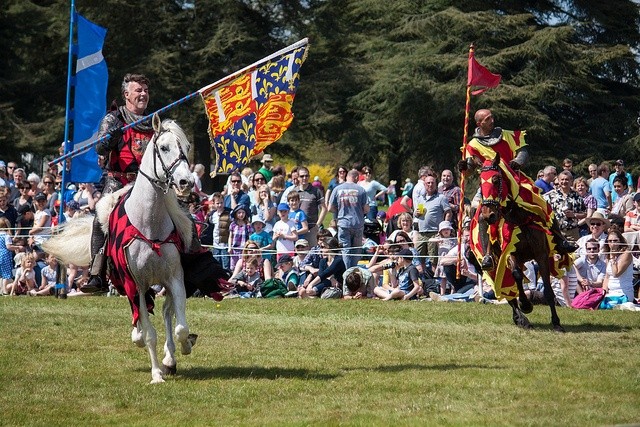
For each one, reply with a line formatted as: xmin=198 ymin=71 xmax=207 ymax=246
xmin=44 ymin=181 xmax=53 ymax=184
xmin=232 ymin=179 xmax=241 ymax=182
xmin=364 ymin=171 xmax=371 ymax=174
xmin=606 ymin=238 xmax=619 ymax=242
xmin=255 ymin=177 xmax=265 ymax=181
xmin=587 ymin=247 xmax=597 ymax=249
xmin=300 ymin=174 xmax=310 ymax=177
xmin=394 ymin=239 xmax=406 ymax=244
xmin=339 ymin=170 xmax=345 ymax=172
xmin=550 ymin=172 xmax=557 ymax=175
xmin=590 ymin=222 xmax=602 ymax=226
xmin=55 ymin=181 xmax=62 ymax=184
xmin=8 ymin=166 xmax=17 ymax=169
xmin=21 ymin=186 xmax=30 ymax=189
xmin=321 ymin=245 xmax=325 ymax=248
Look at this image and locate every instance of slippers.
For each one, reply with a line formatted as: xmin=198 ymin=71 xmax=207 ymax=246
xmin=571 ymin=287 xmax=606 ymax=310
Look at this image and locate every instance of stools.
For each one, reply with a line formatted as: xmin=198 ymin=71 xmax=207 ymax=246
xmin=107 ymin=171 xmax=137 ymax=180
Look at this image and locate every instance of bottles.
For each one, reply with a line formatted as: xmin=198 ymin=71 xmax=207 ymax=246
xmin=615 ymin=159 xmax=624 ymax=164
xmin=54 ymin=199 xmax=66 ymax=207
xmin=67 ymin=200 xmax=81 ymax=210
xmin=438 ymin=220 xmax=455 ymax=234
xmin=633 ymin=192 xmax=640 ymax=201
xmin=377 ymin=209 xmax=386 ymax=218
xmin=261 ymin=154 xmax=273 ymax=162
xmin=251 ymin=215 xmax=266 ymax=227
xmin=277 ymin=202 xmax=290 ymax=210
xmin=34 ymin=192 xmax=47 ymax=200
xmin=395 ymin=248 xmax=413 ymax=260
xmin=295 ymin=238 xmax=309 ymax=247
xmin=15 ymin=203 xmax=30 ymax=221
xmin=585 ymin=211 xmax=606 ymax=226
xmin=275 ymin=254 xmax=293 ymax=267
xmin=230 ymin=204 xmax=251 ymax=219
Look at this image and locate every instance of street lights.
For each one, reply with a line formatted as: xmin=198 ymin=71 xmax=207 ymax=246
xmin=320 ymin=285 xmax=343 ymax=299
xmin=258 ymin=276 xmax=288 ymax=298
xmin=600 ymin=294 xmax=628 ymax=309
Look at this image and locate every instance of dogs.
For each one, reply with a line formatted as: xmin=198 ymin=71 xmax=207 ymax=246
xmin=468 ymin=151 xmax=566 ymax=335
xmin=39 ymin=111 xmax=203 ymax=385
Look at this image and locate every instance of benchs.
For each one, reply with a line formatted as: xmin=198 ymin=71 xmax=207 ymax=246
xmin=67 ymin=14 xmax=108 ymax=187
xmin=198 ymin=34 xmax=309 ymax=177
xmin=467 ymin=48 xmax=503 ymax=98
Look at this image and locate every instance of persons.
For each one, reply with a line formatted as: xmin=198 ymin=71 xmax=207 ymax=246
xmin=458 ymin=109 xmax=580 ymax=271
xmin=535 ymin=157 xmax=639 ymax=311
xmin=80 ymin=75 xmax=208 ymax=293
xmin=0 ymin=140 xmax=107 ymax=300
xmin=518 ymin=261 xmax=546 ymax=306
xmin=190 ymin=152 xmax=485 ymax=306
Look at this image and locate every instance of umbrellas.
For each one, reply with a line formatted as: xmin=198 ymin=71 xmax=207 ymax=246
xmin=482 ymin=256 xmax=497 ymax=268
xmin=556 ymin=240 xmax=579 ymax=255
xmin=80 ymin=276 xmax=109 ymax=293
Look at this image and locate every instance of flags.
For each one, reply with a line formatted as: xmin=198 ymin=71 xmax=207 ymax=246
xmin=467 ymin=48 xmax=503 ymax=98
xmin=67 ymin=14 xmax=108 ymax=187
xmin=198 ymin=34 xmax=309 ymax=177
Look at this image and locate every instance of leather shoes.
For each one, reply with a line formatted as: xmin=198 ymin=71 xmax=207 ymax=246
xmin=458 ymin=159 xmax=468 ymax=173
xmin=510 ymin=160 xmax=522 ymax=170
xmin=107 ymin=123 xmax=126 ymax=138
